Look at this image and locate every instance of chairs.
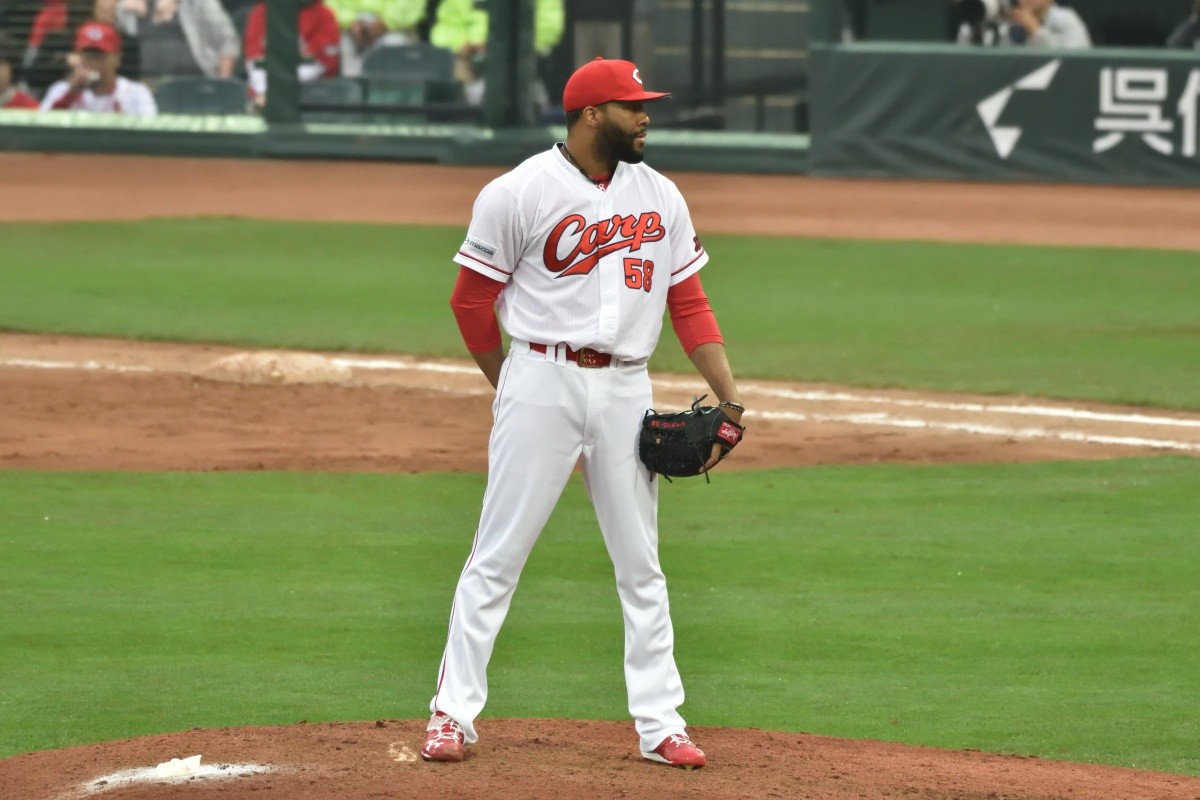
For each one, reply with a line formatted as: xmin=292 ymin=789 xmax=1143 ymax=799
xmin=2 ymin=0 xmax=499 ymax=128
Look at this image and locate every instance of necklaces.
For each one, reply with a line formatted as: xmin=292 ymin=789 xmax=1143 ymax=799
xmin=563 ymin=143 xmax=614 ymax=183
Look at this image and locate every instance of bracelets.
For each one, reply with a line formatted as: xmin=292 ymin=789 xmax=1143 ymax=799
xmin=719 ymin=401 xmax=745 ymax=413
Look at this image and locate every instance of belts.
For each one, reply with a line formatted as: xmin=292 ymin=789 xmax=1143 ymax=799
xmin=529 ymin=342 xmax=611 ymax=368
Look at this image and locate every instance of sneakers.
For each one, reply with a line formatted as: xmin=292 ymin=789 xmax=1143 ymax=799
xmin=640 ymin=734 xmax=707 ymax=766
xmin=422 ymin=711 xmax=463 ymax=761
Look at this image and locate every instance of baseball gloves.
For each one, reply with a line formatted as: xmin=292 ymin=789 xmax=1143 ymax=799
xmin=639 ymin=407 xmax=743 ymax=477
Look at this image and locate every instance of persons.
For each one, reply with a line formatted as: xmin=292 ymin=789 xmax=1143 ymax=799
xmin=0 ymin=56 xmax=38 ymax=109
xmin=419 ymin=58 xmax=746 ymax=771
xmin=955 ymin=0 xmax=1094 ymax=50
xmin=241 ymin=0 xmax=341 ymax=111
xmin=114 ymin=0 xmax=241 ymax=80
xmin=34 ymin=20 xmax=159 ymax=115
xmin=338 ymin=12 xmax=408 ymax=76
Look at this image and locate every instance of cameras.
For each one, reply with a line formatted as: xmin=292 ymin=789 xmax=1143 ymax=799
xmin=962 ymin=0 xmax=1019 ymax=25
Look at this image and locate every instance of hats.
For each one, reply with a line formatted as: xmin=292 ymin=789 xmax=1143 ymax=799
xmin=563 ymin=57 xmax=671 ymax=111
xmin=76 ymin=23 xmax=119 ymax=52
